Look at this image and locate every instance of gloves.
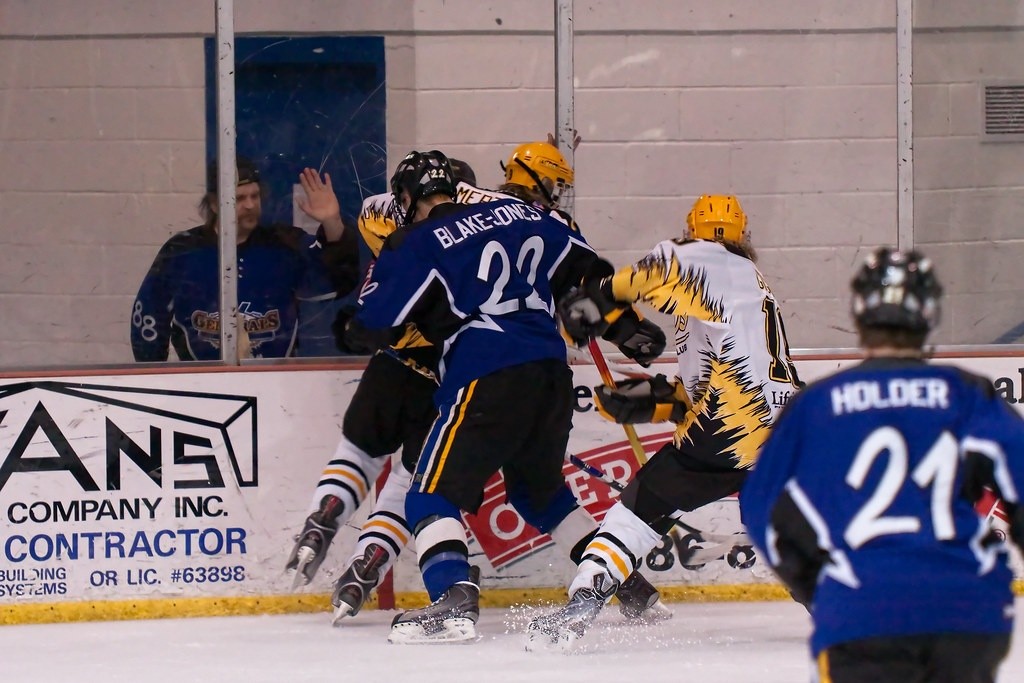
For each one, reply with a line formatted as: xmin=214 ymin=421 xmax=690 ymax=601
xmin=619 ymin=319 xmax=667 ymax=368
xmin=594 ymin=367 xmax=687 ymax=424
xmin=561 ymin=282 xmax=612 ymax=342
xmin=331 ymin=305 xmax=360 ymax=352
xmin=357 ymin=324 xmax=408 ymax=352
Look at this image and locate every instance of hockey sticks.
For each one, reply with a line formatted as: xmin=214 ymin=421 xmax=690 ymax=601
xmin=583 ymin=329 xmax=735 ymax=566
xmin=344 ymin=318 xmax=756 ymax=546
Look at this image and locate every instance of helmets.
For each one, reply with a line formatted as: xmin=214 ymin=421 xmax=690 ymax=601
xmin=445 ymin=157 xmax=476 ymax=187
xmin=850 ymin=245 xmax=940 ymax=335
xmin=391 ymin=150 xmax=457 ymax=198
xmin=505 ymin=142 xmax=574 ymax=189
xmin=687 ymin=192 xmax=748 ymax=246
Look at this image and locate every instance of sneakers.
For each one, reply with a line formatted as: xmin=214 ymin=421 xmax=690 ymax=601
xmin=330 ymin=543 xmax=390 ymax=626
xmin=285 ymin=494 xmax=344 ymax=589
xmin=388 ymin=566 xmax=481 ymax=644
xmin=525 ymin=589 xmax=605 ymax=651
xmin=619 ymin=570 xmax=675 ymax=626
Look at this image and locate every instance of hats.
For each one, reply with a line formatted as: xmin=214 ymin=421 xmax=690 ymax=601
xmin=207 ymin=154 xmax=258 ymax=189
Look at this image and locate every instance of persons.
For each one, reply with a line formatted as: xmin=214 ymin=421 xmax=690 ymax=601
xmin=527 ymin=192 xmax=816 ymax=637
xmin=286 ymin=139 xmax=665 ymax=616
xmin=737 ymin=245 xmax=1024 ymax=683
xmin=129 ymin=159 xmax=362 ymax=365
xmin=330 ymin=149 xmax=666 ymax=644
